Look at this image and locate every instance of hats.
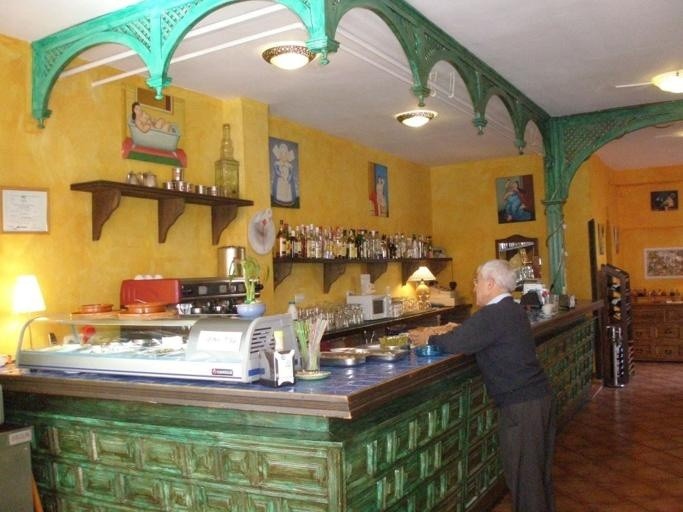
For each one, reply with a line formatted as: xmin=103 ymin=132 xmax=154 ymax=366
xmin=247 ymin=210 xmax=276 ymax=255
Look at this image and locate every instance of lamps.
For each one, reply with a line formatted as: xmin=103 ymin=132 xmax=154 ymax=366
xmin=261 ymin=45 xmax=316 ymax=71
xmin=407 ymin=266 xmax=437 ymax=310
xmin=12 ymin=274 xmax=47 ymax=349
xmin=652 ymin=71 xmax=683 ymax=94
xmin=397 ymin=111 xmax=435 ymax=129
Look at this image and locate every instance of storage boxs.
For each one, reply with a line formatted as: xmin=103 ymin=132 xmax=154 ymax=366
xmin=0 ymin=423 xmax=34 ymax=512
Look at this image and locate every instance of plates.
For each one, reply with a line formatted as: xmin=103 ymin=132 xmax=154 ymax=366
xmin=320 ymin=343 xmax=442 ymax=368
xmin=81 ymin=303 xmax=167 ymax=313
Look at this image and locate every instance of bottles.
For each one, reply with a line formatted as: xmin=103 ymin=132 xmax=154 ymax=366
xmin=273 ymin=219 xmax=434 ymax=259
xmin=214 ymin=123 xmax=241 ymax=200
xmin=288 ymin=301 xmax=297 ymax=321
xmin=127 ymin=170 xmax=157 ymax=188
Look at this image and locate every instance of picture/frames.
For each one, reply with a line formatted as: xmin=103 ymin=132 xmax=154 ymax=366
xmin=644 ymin=247 xmax=683 ymax=279
xmin=0 ymin=185 xmax=51 ymax=234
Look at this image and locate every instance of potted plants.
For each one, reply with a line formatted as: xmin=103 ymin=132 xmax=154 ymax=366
xmin=227 ymin=256 xmax=270 ymax=318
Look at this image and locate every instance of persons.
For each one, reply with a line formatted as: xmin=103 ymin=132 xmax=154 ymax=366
xmin=408 ymin=258 xmax=562 ymax=511
xmin=375 ymin=176 xmax=385 ymax=216
xmin=272 ymin=143 xmax=295 ymax=207
xmin=130 ymin=102 xmax=172 ymax=134
xmin=504 ymin=180 xmax=523 ymax=222
xmin=502 ymin=179 xmax=528 ymax=209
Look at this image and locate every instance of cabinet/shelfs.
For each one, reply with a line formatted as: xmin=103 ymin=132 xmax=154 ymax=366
xmin=605 ymin=263 xmax=636 ymax=382
xmin=272 ymin=257 xmax=473 ymax=351
xmin=630 ymin=296 xmax=683 ymax=363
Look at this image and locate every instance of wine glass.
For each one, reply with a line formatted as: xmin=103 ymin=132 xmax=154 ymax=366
xmin=417 ymin=296 xmax=431 ymax=313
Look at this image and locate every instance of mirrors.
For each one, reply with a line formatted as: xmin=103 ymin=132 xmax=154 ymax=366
xmin=495 ymin=234 xmax=542 ymax=292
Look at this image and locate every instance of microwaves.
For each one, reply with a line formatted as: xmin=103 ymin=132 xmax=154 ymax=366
xmin=347 ymin=295 xmax=391 ymax=320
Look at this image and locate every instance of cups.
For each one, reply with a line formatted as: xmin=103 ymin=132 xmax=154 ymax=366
xmin=299 ymin=303 xmax=364 ymax=331
xmin=300 ymin=345 xmax=320 ymax=373
xmin=166 ymin=167 xmax=217 ymax=196
